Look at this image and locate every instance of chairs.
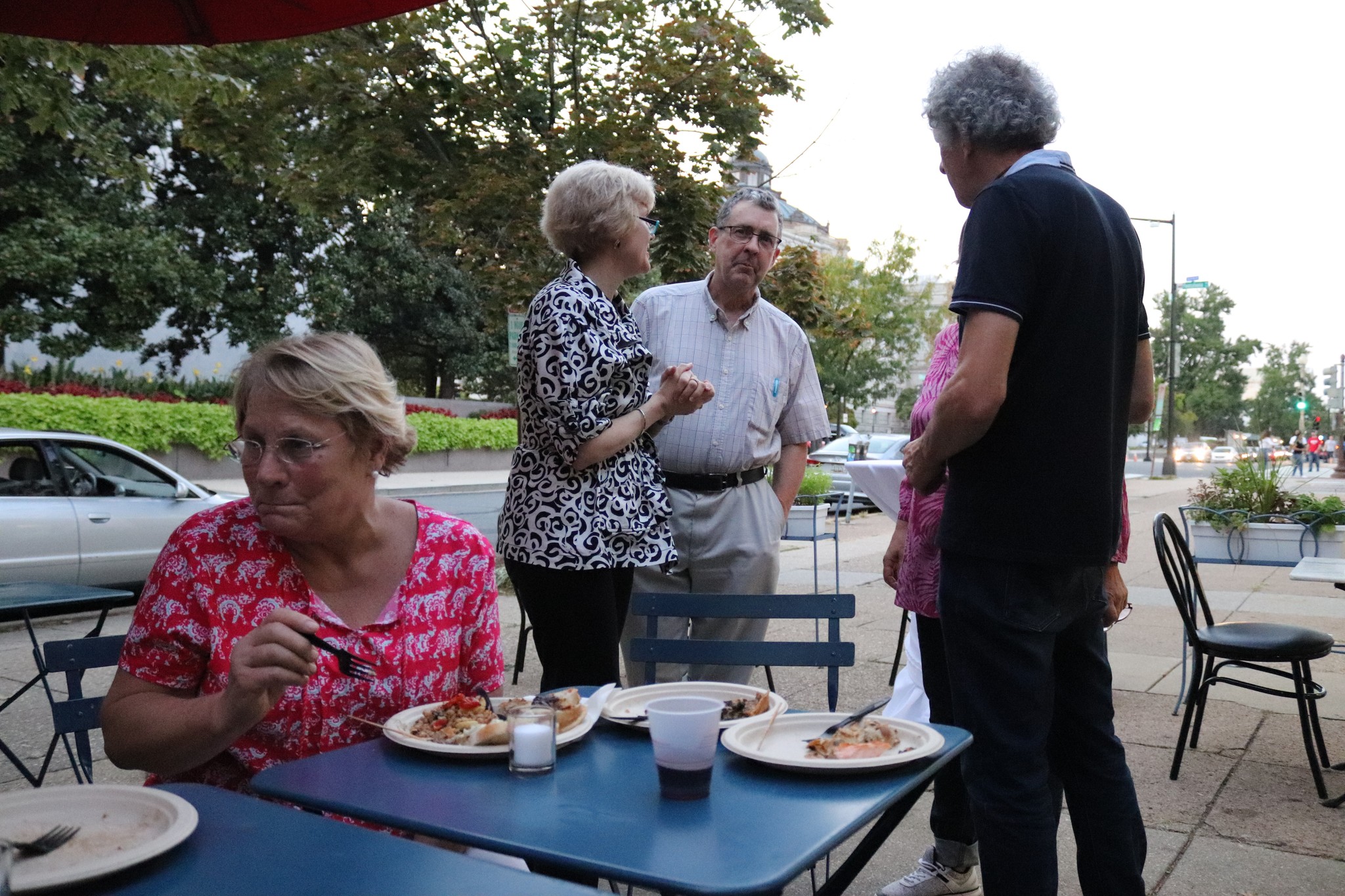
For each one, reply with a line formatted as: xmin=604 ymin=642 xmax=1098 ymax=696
xmin=627 ymin=590 xmax=858 ymax=711
xmin=1153 ymin=513 xmax=1334 ymax=799
xmin=42 ymin=634 xmax=127 ymax=785
xmin=8 ymin=457 xmax=36 ymax=482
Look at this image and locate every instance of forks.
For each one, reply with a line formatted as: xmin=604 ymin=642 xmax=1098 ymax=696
xmin=298 ymin=629 xmax=379 ymax=685
xmin=0 ymin=824 xmax=82 ymax=856
xmin=801 ymin=696 xmax=892 ymax=742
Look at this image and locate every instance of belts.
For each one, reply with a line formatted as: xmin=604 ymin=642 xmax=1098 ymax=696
xmin=660 ymin=465 xmax=765 ymax=493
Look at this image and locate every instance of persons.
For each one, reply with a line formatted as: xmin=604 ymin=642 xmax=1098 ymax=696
xmin=619 ymin=186 xmax=831 ymax=687
xmin=901 ymin=49 xmax=1155 ymax=896
xmin=1258 ymin=430 xmax=1335 ymax=477
xmin=99 ymin=330 xmax=506 ymax=855
xmin=879 ymin=319 xmax=1129 ymax=896
xmin=494 ymin=160 xmax=717 ymax=697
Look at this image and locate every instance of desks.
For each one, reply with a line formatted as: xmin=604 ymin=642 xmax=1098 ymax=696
xmin=1288 ymin=557 xmax=1345 ymax=809
xmin=251 ymin=686 xmax=973 ymax=896
xmin=845 ymin=460 xmax=929 ymax=722
xmin=10 ymin=782 xmax=614 ymax=896
xmin=0 ymin=578 xmax=137 ymax=788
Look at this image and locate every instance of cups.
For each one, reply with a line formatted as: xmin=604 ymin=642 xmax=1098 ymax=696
xmin=645 ymin=697 xmax=725 ymax=799
xmin=507 ymin=705 xmax=556 ymax=772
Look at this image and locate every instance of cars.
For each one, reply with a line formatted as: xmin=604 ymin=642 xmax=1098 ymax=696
xmin=807 ymin=424 xmax=910 ymax=512
xmin=0 ymin=430 xmax=245 ymax=589
xmin=1176 ymin=442 xmax=1289 ymax=463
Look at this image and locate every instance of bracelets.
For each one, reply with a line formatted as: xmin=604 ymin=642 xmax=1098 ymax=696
xmin=631 ymin=408 xmax=646 ymax=438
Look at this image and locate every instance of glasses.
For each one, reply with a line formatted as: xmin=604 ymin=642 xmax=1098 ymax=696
xmin=222 ymin=431 xmax=349 ymax=466
xmin=717 ymin=225 xmax=782 ymax=251
xmin=634 ymin=214 xmax=660 ymax=236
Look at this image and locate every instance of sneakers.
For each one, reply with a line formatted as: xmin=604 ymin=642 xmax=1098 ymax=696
xmin=874 ymin=845 xmax=982 ymax=896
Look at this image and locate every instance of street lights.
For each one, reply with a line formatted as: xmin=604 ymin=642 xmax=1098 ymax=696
xmin=1297 ymin=354 xmax=1307 ymax=454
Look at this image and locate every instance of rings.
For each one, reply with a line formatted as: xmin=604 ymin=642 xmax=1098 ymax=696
xmin=689 ymin=377 xmax=699 ymax=386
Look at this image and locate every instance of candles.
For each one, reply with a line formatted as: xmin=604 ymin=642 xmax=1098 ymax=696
xmin=513 ymin=725 xmax=554 ymax=765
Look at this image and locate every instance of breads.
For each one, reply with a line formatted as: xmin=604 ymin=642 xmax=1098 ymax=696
xmin=473 ymin=688 xmax=587 ymax=745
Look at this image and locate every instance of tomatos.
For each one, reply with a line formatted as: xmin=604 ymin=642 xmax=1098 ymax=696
xmin=431 ymin=693 xmax=480 ymax=731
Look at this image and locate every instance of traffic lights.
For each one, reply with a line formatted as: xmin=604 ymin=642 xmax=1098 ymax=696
xmin=1323 ymin=366 xmax=1337 ymax=397
xmin=1312 ymin=417 xmax=1321 ymax=431
xmin=1296 ymin=391 xmax=1303 ymax=410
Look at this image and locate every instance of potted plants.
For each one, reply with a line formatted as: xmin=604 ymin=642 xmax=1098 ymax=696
xmin=767 ymin=463 xmax=835 ymax=537
xmin=1182 ymin=397 xmax=1345 ymax=566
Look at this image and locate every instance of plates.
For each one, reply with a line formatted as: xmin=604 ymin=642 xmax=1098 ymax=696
xmin=721 ymin=711 xmax=946 ymax=768
xmin=381 ymin=695 xmax=595 ymax=755
xmin=0 ymin=783 xmax=199 ymax=892
xmin=601 ymin=681 xmax=789 ymax=728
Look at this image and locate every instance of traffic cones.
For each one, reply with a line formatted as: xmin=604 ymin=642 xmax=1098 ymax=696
xmin=1133 ymin=453 xmax=1138 ymax=462
